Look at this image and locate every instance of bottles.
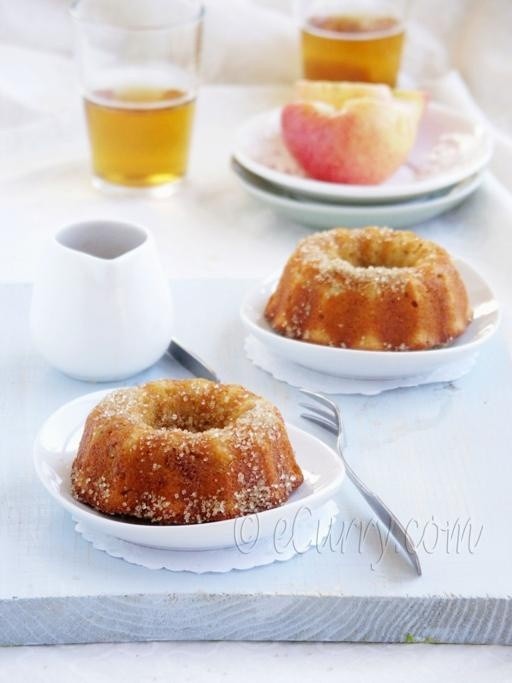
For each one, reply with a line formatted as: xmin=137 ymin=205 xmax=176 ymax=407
xmin=27 ymin=216 xmax=173 ymax=383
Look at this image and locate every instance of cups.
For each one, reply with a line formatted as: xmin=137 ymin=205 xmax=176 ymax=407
xmin=70 ymin=0 xmax=203 ymax=205
xmin=300 ymin=10 xmax=405 ymax=88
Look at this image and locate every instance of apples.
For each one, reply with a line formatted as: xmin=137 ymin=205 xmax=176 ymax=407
xmin=281 ymin=78 xmax=421 ymax=186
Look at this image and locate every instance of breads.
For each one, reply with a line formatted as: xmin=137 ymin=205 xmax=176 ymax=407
xmin=264 ymin=225 xmax=474 ymax=351
xmin=71 ymin=378 xmax=304 ymax=525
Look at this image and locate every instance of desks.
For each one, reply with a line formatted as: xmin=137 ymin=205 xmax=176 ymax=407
xmin=0 ymin=1 xmax=510 ymax=682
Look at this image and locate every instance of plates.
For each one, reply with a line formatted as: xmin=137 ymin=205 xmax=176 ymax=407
xmin=223 ymin=102 xmax=490 ymax=232
xmin=239 ymin=271 xmax=500 ymax=379
xmin=61 ymin=419 xmax=346 ymax=549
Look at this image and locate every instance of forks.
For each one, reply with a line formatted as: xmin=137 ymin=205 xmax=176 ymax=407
xmin=296 ymin=384 xmax=421 ymax=576
